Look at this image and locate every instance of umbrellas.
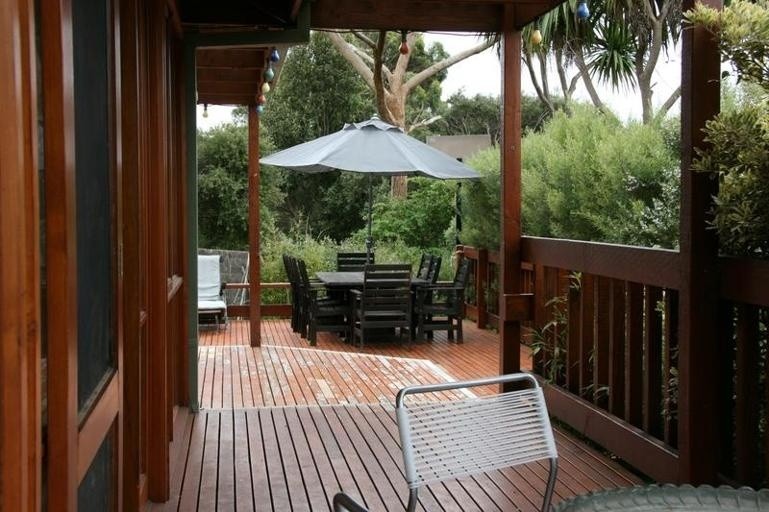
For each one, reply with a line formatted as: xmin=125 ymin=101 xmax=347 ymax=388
xmin=258 ymin=112 xmax=485 ymax=266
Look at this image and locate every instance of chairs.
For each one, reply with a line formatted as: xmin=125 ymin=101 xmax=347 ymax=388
xmin=281 ymin=252 xmax=471 ymax=351
xmin=198 ymin=254 xmax=231 ymax=333
xmin=333 ymin=375 xmax=560 ymax=512
